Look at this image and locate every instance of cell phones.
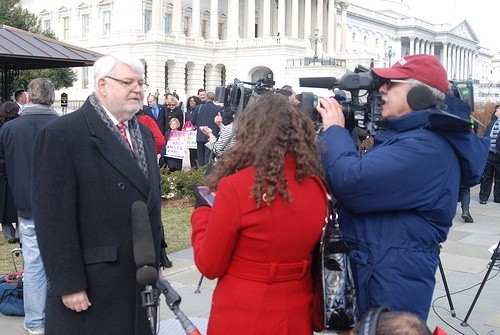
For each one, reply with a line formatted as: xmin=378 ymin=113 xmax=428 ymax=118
xmin=197 ymin=186 xmax=216 ymax=207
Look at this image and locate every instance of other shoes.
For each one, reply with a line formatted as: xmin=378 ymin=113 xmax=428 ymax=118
xmin=24 ymin=319 xmax=44 ymax=334
xmin=162 ymin=192 xmax=174 ymax=199
xmin=462 ymin=209 xmax=473 ymax=223
xmin=480 ymin=200 xmax=486 ymax=204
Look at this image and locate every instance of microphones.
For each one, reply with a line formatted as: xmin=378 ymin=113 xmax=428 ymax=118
xmin=131 ymin=200 xmax=159 ymax=285
xmin=299 ymin=77 xmax=339 ymax=89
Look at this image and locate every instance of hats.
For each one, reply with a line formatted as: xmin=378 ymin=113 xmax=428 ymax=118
xmin=371 ymin=54 xmax=449 ymax=97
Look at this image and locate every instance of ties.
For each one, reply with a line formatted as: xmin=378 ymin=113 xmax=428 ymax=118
xmin=116 ymin=123 xmax=131 ymax=151
xmin=153 ymin=108 xmax=157 ymax=121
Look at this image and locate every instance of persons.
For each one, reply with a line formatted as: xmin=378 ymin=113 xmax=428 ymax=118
xmin=315 ymin=54 xmax=492 ymax=335
xmin=0 ymin=77 xmax=60 ymax=335
xmin=15 ymin=90 xmax=27 ymax=115
xmin=143 ymin=89 xmax=253 ymax=176
xmin=458 ymin=187 xmax=474 ymax=223
xmin=0 ymin=101 xmax=19 ymax=127
xmin=191 ymin=93 xmax=334 ymax=335
xmin=30 ymin=52 xmax=173 ymax=335
xmin=347 ymin=310 xmax=432 ymax=335
xmin=479 ymin=104 xmax=500 ymax=204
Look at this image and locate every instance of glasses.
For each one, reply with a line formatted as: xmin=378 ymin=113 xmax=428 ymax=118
xmin=380 ymin=77 xmax=421 ymax=91
xmin=147 ymin=101 xmax=154 ymax=103
xmin=189 ymin=100 xmax=195 ymax=103
xmin=104 ymin=75 xmax=149 ymax=92
xmin=168 ymin=104 xmax=175 ymax=107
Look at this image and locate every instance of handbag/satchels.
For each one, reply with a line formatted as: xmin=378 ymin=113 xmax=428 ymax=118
xmin=310 ymin=173 xmax=358 ymax=330
xmin=0 ymin=277 xmax=25 ymax=316
